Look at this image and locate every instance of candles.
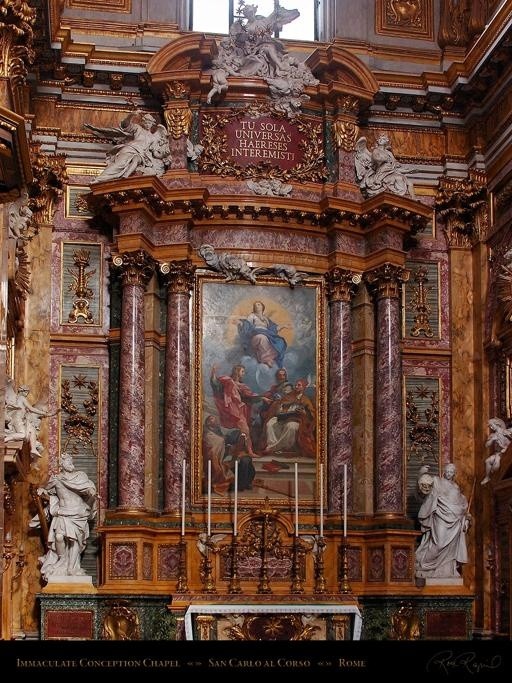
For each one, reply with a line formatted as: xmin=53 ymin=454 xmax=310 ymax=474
xmin=208 ymin=460 xmax=211 ymax=537
xmin=343 ymin=463 xmax=348 ymax=537
xmin=233 ymin=460 xmax=238 ymax=537
xmin=319 ymin=463 xmax=324 ymax=537
xmin=181 ymin=459 xmax=187 ymax=536
xmin=294 ymin=462 xmax=299 ymax=537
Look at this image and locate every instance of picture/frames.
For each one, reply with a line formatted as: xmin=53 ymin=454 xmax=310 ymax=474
xmin=189 ymin=272 xmax=329 ymax=512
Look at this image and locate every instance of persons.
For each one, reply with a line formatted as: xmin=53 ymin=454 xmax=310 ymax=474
xmin=414 ymin=463 xmax=471 ymax=585
xmin=232 ymin=301 xmax=289 ymax=367
xmin=187 ymin=139 xmax=204 ymax=172
xmin=206 ymin=4 xmax=319 ymax=118
xmin=29 ymin=452 xmax=102 ymax=577
xmin=480 ymin=417 xmax=512 ymax=484
xmin=4 ymin=377 xmax=58 ymax=458
xmin=355 ymin=135 xmax=419 ymax=199
xmin=92 ymin=109 xmax=171 ymax=182
xmin=201 ymin=364 xmax=317 ymax=496
